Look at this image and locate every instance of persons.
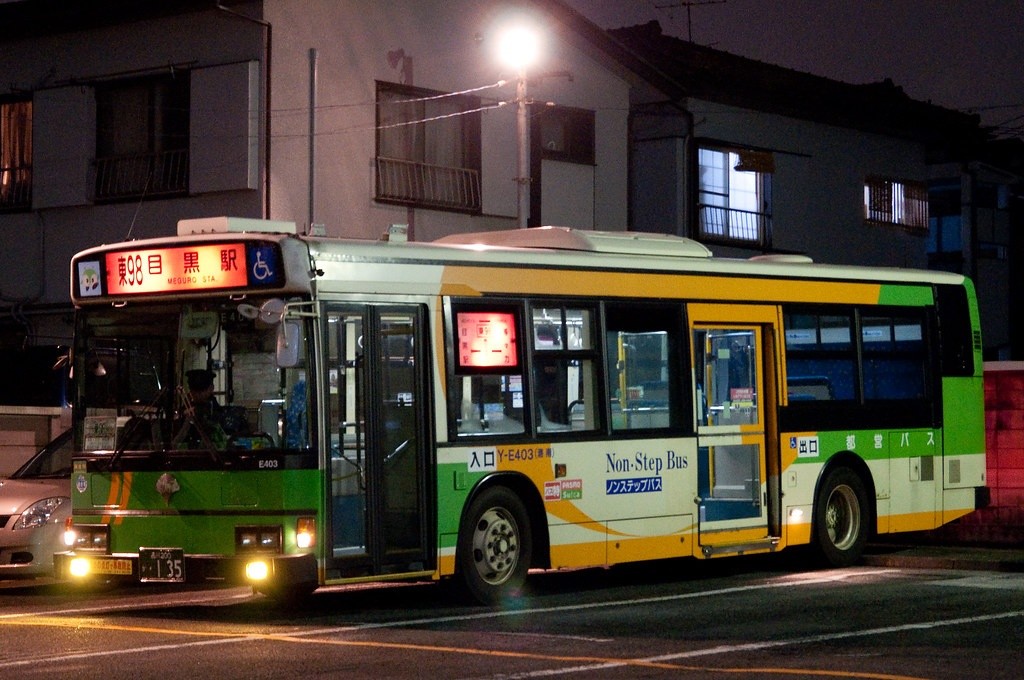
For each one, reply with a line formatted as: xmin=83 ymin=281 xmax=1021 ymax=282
xmin=180 ymin=369 xmax=227 ymax=450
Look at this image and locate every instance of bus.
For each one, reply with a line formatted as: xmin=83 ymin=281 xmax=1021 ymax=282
xmin=52 ymin=217 xmax=991 ymax=610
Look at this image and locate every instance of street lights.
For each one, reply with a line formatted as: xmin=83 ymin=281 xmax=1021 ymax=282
xmin=499 ymin=17 xmax=546 ymax=231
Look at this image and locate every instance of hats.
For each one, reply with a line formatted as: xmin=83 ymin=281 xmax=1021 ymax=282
xmin=184 ymin=369 xmax=217 ymax=387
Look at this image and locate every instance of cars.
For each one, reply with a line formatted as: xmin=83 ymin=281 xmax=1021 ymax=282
xmin=0 ymin=427 xmax=123 ymax=594
